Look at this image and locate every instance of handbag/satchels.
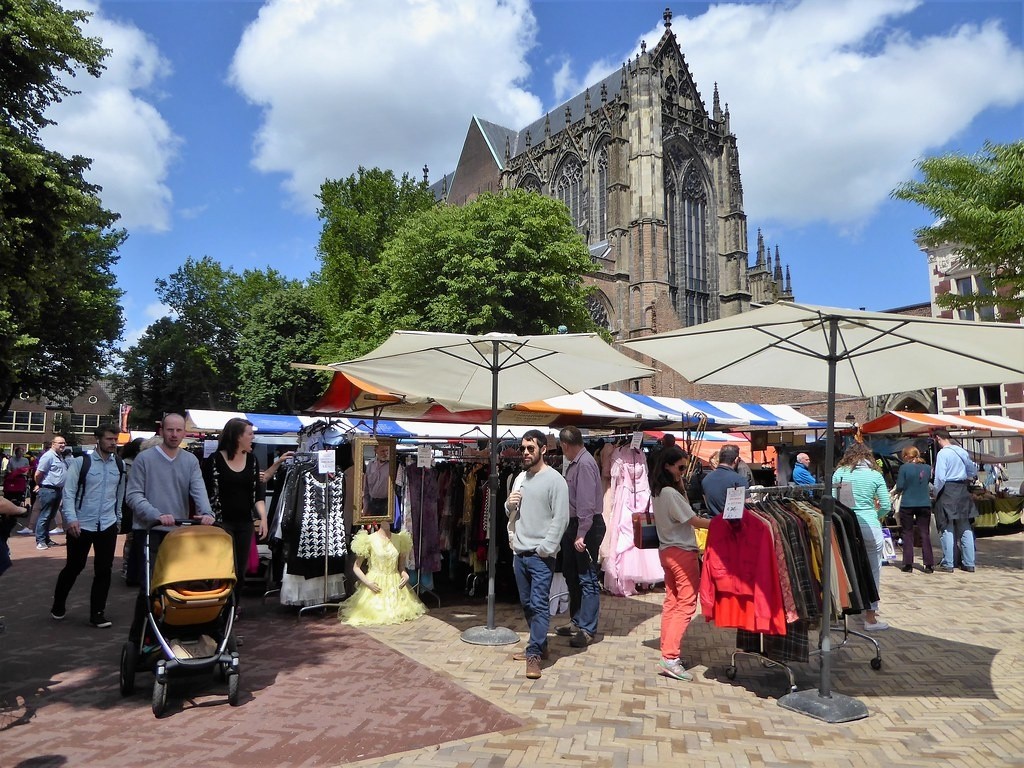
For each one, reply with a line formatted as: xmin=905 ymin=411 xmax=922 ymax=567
xmin=634 ymin=492 xmax=659 ymax=549
xmin=507 ymin=471 xmax=527 ymax=551
xmin=207 ymin=456 xmax=222 ymax=523
xmin=247 ymin=530 xmax=259 ymax=574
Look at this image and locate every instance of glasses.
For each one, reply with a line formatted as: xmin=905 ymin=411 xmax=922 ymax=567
xmin=672 ymin=464 xmax=687 ymax=471
xmin=519 ymin=445 xmax=535 ymax=452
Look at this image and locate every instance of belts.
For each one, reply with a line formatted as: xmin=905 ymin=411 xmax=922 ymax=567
xmin=40 ymin=484 xmax=62 ymax=491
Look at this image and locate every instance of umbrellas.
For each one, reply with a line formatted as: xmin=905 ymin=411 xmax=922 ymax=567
xmin=615 ymin=299 xmax=1024 ymax=724
xmin=327 ymin=329 xmax=663 ymax=646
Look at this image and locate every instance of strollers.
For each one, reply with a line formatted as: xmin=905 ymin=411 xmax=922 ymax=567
xmin=3 ymin=466 xmax=33 ymax=518
xmin=120 ymin=519 xmax=240 ymax=717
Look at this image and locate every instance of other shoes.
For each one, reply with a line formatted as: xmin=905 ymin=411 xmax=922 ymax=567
xmin=942 ymin=567 xmax=953 ymax=573
xmin=924 ymin=564 xmax=934 ymax=573
xmin=901 ymin=564 xmax=912 ymax=572
xmin=121 ymin=568 xmax=128 ymax=579
xmin=960 ymin=565 xmax=974 ymax=572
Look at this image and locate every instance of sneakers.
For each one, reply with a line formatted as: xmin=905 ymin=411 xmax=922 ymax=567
xmin=658 ymin=656 xmax=693 ymax=682
xmin=46 ymin=540 xmax=56 ymax=545
xmin=36 ymin=541 xmax=48 ymax=550
xmin=52 ymin=598 xmax=65 ymax=619
xmin=49 ymin=527 xmax=64 ymax=534
xmin=512 ymin=645 xmax=549 ymax=660
xmin=90 ymin=610 xmax=112 ymax=627
xmin=571 ymin=632 xmax=593 ymax=647
xmin=526 ymin=655 xmax=541 ymax=679
xmin=556 ymin=619 xmax=581 ymax=636
xmin=17 ymin=526 xmax=33 ymax=533
xmin=864 ymin=620 xmax=889 ymax=631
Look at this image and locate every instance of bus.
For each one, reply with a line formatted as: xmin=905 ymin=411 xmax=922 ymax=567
xmin=203 ymin=432 xmax=443 ymax=560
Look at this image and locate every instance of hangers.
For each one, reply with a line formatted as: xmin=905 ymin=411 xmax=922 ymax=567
xmin=744 ymin=482 xmax=828 ymax=514
xmin=286 ymin=452 xmax=342 ymax=476
xmin=296 ymin=412 xmax=377 ymax=437
xmin=432 ymin=430 xmax=639 ymax=475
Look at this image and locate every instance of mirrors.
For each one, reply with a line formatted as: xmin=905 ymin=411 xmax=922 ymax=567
xmin=353 ymin=437 xmax=397 ymax=525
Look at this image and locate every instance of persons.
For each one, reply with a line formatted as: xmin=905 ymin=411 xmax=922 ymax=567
xmin=49 ymin=424 xmax=127 ymax=628
xmin=201 ymin=418 xmax=268 ymax=622
xmin=263 ymin=444 xmax=298 ymax=581
xmin=702 ymin=444 xmax=756 ymax=518
xmin=896 ymin=445 xmax=935 ymax=574
xmin=363 ymin=444 xmax=388 ymax=516
xmin=831 ymin=437 xmax=892 ymax=631
xmin=793 ymin=452 xmax=817 ymax=499
xmin=125 ymin=412 xmax=216 ymax=642
xmin=555 ymin=426 xmax=607 ymax=647
xmin=336 ymin=520 xmax=431 ymax=630
xmin=646 ymin=444 xmax=711 ymax=683
xmin=0 ymin=435 xmax=85 ymax=576
xmin=504 ymin=429 xmax=571 ymax=679
xmin=932 ymin=428 xmax=980 ymax=573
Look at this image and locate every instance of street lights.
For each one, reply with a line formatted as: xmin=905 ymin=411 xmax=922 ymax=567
xmin=976 ymin=438 xmax=985 ymax=471
